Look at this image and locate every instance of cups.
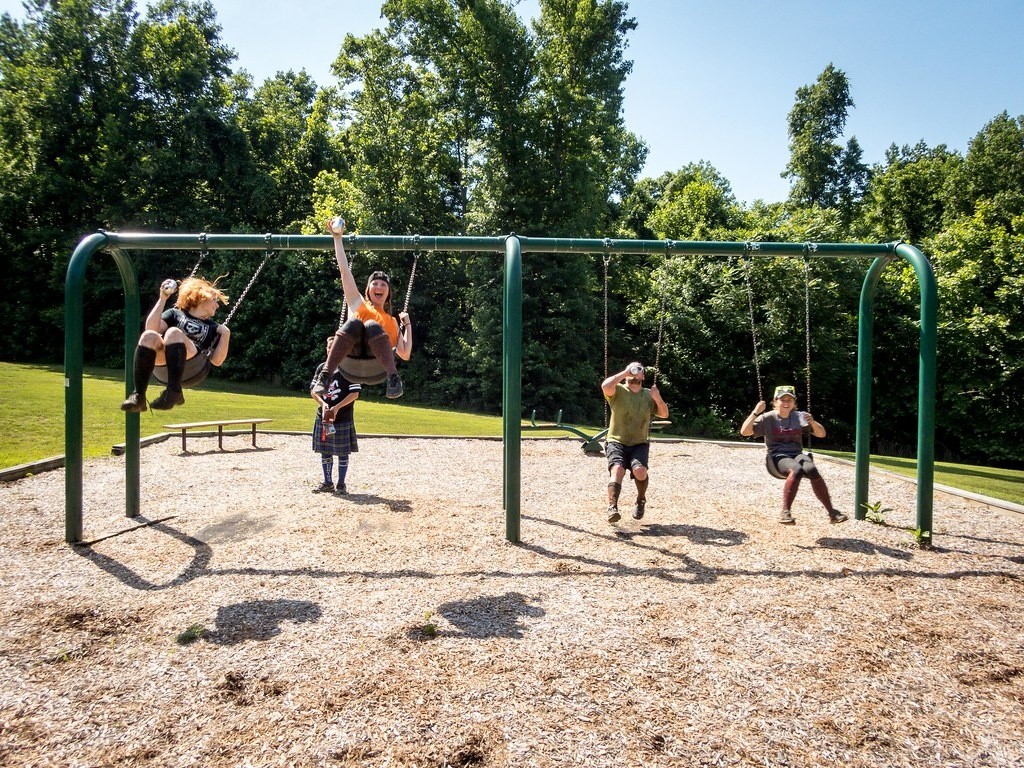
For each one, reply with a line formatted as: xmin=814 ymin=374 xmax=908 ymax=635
xmin=164 ymin=280 xmax=177 ymax=294
xmin=799 ymin=413 xmax=809 ymax=427
xmin=324 ymin=409 xmax=334 ymax=423
xmin=332 ymin=218 xmax=343 ymax=233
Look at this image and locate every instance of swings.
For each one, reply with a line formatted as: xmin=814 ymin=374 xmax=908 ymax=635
xmin=743 ymin=256 xmax=814 ymax=479
xmin=602 ymin=252 xmax=674 ymax=458
xmin=151 ymin=249 xmax=277 ymax=386
xmin=336 ymin=250 xmax=423 ymax=386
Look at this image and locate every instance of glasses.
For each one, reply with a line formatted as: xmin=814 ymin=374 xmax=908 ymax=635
xmin=637 ymin=366 xmax=643 ymax=373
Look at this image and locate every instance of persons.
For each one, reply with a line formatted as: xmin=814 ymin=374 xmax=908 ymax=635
xmin=740 ymin=385 xmax=849 ymax=524
xmin=601 ymin=361 xmax=669 ymax=522
xmin=120 ymin=275 xmax=231 ymax=412
xmin=313 ymin=216 xmax=411 ymax=398
xmin=309 ymin=335 xmax=362 ymax=494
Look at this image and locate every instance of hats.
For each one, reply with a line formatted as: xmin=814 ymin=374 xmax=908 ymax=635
xmin=367 ymin=270 xmax=391 ymax=285
xmin=774 ymin=386 xmax=797 ymax=400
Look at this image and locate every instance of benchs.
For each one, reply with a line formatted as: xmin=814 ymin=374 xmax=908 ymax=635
xmin=163 ymin=418 xmax=274 ymax=451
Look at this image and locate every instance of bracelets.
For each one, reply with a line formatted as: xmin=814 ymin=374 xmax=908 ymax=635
xmin=404 ymin=323 xmax=411 ymax=328
xmin=752 ymin=410 xmax=758 ymax=417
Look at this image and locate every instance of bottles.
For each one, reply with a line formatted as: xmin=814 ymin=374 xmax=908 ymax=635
xmin=321 ymin=424 xmax=326 ymax=441
xmin=328 ymin=424 xmax=336 ymax=434
xmin=323 ymin=424 xmax=330 ymax=435
xmin=631 ymin=366 xmax=642 ymax=374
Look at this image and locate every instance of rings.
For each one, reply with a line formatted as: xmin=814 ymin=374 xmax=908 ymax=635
xmin=809 ymin=414 xmax=811 ymax=416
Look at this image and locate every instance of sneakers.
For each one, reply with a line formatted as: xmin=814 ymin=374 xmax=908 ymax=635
xmin=829 ymin=510 xmax=848 ymax=524
xmin=386 ymin=373 xmax=404 ymax=399
xmin=150 ymin=389 xmax=185 ymax=409
xmin=633 ymin=497 xmax=646 ymax=519
xmin=312 ymin=482 xmax=335 ymax=494
xmin=608 ymin=508 xmax=621 ymax=522
xmin=776 ymin=510 xmax=797 ymax=525
xmin=335 ymin=483 xmax=346 ymax=494
xmin=121 ymin=392 xmax=154 ymax=416
xmin=313 ymin=371 xmax=333 ymax=395
